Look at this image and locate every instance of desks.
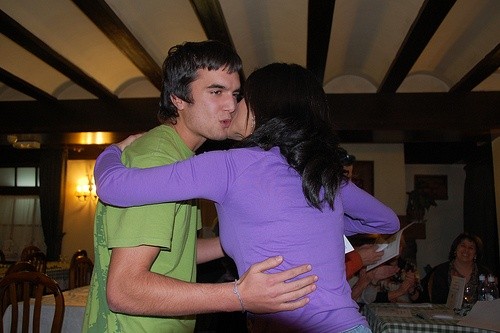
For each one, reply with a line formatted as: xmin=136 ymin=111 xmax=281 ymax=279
xmin=40 ymin=261 xmax=70 ymax=296
xmin=2 ymin=285 xmax=90 ymax=333
xmin=364 ymin=301 xmax=500 ymax=333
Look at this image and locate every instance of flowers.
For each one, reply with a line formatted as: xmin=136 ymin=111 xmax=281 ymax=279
xmin=387 ymin=258 xmax=415 ymax=282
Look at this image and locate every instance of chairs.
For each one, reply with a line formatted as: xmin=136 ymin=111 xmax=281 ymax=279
xmin=0 ymin=246 xmax=90 ymax=333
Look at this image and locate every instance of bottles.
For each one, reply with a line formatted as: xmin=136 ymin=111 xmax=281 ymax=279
xmin=485 ymin=275 xmax=499 ymax=301
xmin=478 ymin=274 xmax=488 ymax=300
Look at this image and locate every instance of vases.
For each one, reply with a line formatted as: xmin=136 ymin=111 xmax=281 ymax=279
xmin=390 ymin=278 xmax=411 ymax=302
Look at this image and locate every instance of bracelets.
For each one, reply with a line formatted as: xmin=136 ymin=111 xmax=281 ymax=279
xmin=233 ymin=279 xmax=245 ymax=313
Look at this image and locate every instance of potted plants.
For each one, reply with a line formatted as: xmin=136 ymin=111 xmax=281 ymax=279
xmin=407 ymin=184 xmax=438 ymax=220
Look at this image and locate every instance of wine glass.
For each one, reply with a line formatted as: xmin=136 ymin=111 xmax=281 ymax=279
xmin=60 ymin=255 xmax=68 ymax=264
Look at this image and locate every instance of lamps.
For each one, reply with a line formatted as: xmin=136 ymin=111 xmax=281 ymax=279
xmin=76 ymin=177 xmax=98 ymax=202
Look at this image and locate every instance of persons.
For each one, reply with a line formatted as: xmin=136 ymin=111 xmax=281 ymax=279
xmin=82 ymin=40 xmax=319 ymax=333
xmin=346 ymin=232 xmax=412 ymax=304
xmin=94 ymin=62 xmax=400 ymax=333
xmin=378 ymin=233 xmax=422 ymax=302
xmin=420 ymin=232 xmax=490 ymax=304
